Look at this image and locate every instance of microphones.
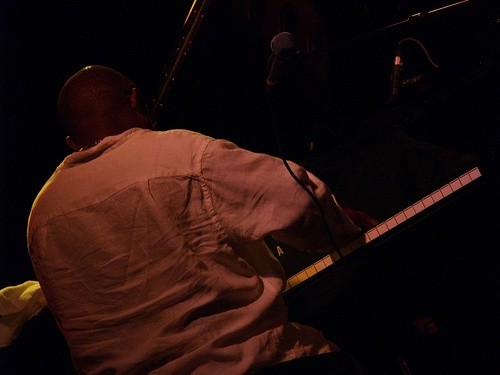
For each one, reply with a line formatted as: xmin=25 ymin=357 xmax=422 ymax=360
xmin=263 ymin=30 xmax=296 ymax=101
xmin=389 ymin=45 xmax=403 ymax=104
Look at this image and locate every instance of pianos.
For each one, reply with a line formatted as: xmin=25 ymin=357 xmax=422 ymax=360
xmin=282 ymin=153 xmax=487 ymax=302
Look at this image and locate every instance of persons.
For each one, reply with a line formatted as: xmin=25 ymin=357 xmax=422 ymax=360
xmin=27 ymin=65 xmax=379 ymax=374
